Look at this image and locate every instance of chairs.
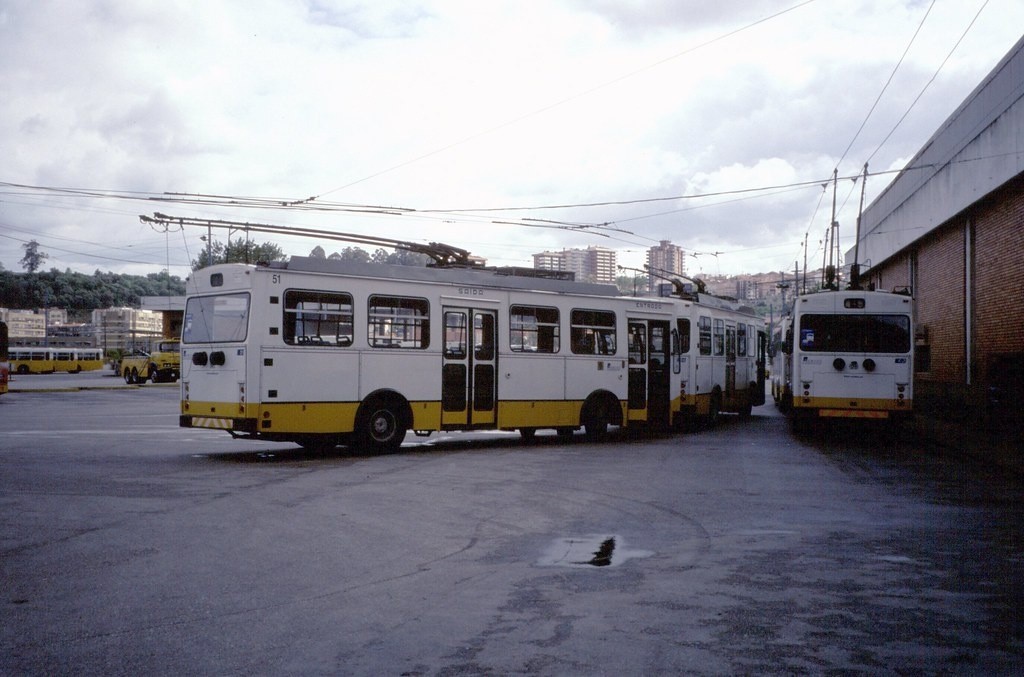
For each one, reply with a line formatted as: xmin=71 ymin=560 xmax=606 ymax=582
xmin=297 ymin=334 xmax=351 ymax=347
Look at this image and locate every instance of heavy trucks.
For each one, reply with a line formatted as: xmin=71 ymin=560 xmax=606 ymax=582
xmin=120 ymin=337 xmax=181 ymax=384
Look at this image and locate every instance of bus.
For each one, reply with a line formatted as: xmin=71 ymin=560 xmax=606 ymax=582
xmin=770 ymin=286 xmax=916 ymax=418
xmin=0 ymin=320 xmax=10 ymax=395
xmin=8 ymin=347 xmax=104 ymax=374
xmin=178 ymin=253 xmax=767 ymax=455
